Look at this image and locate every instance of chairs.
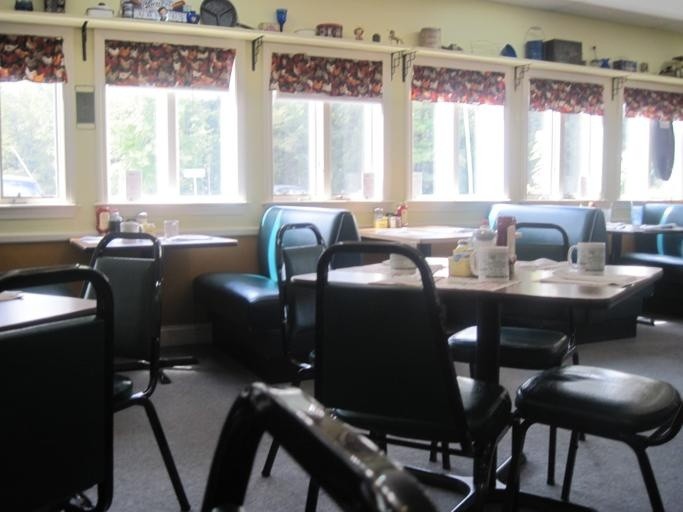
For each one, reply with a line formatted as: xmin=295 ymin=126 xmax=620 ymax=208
xmin=275 ymin=222 xmax=681 ymax=511
xmin=1 ymin=232 xmax=191 ymax=511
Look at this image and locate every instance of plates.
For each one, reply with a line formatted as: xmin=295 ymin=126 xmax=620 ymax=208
xmin=175 ymin=235 xmax=209 ymax=240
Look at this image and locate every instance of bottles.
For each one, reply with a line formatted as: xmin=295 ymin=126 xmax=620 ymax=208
xmin=374 ymin=203 xmax=408 ymax=228
xmin=449 ymin=210 xmax=516 ymax=282
xmin=95 ymin=204 xmax=155 ymax=238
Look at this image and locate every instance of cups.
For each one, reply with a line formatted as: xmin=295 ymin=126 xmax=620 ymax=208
xmin=163 ymin=220 xmax=179 ymax=240
xmin=601 ymin=208 xmax=612 ymax=227
xmin=631 ymin=206 xmax=641 ymax=227
xmin=390 ymin=238 xmax=419 ymax=275
xmin=567 ymin=242 xmax=605 ymax=275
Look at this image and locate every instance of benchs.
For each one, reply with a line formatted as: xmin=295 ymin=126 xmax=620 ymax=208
xmin=2 ymin=201 xmax=683 ymax=382
